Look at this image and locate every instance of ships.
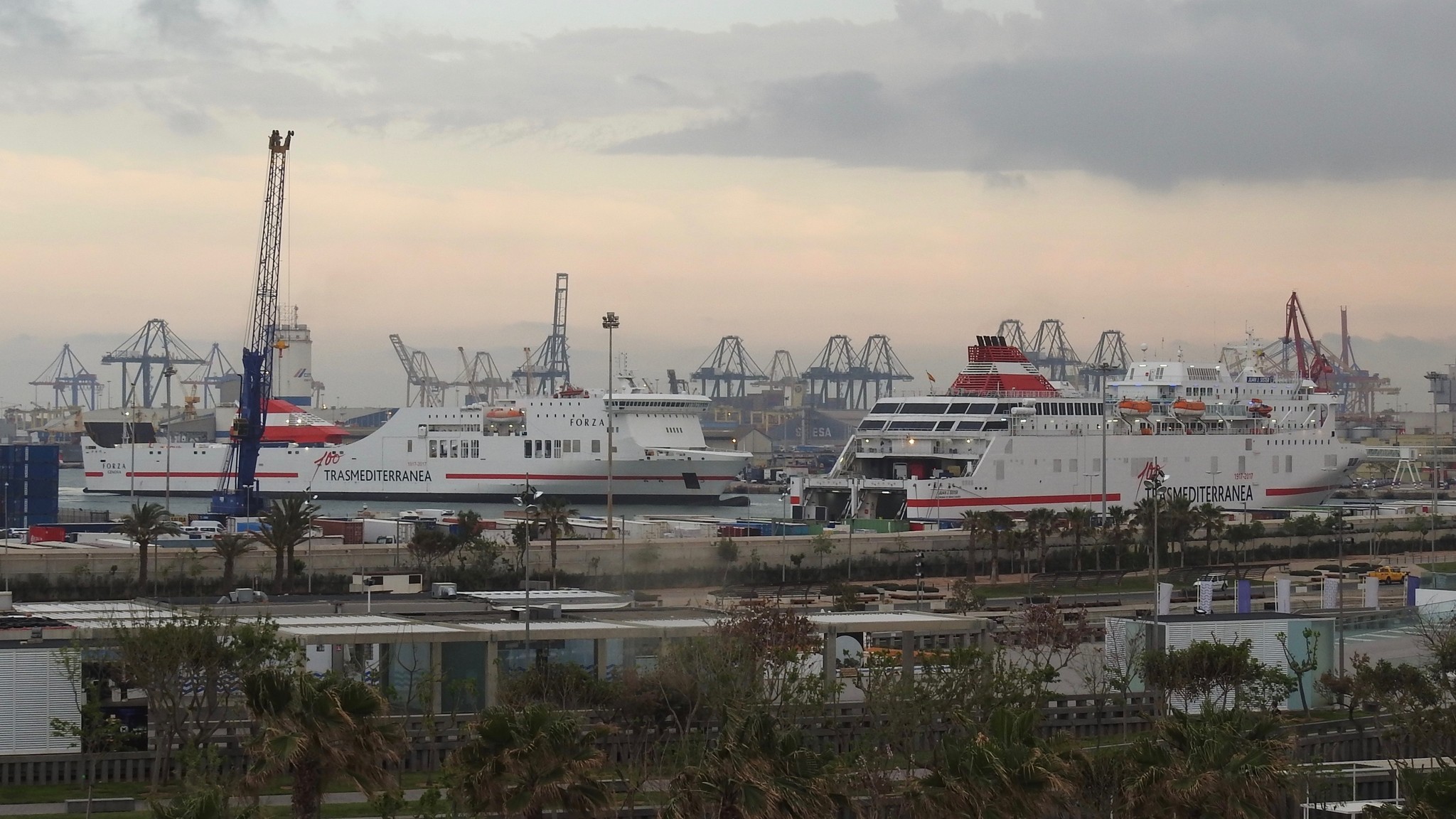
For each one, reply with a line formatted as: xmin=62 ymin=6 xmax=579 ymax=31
xmin=786 ymin=334 xmax=1370 ymax=532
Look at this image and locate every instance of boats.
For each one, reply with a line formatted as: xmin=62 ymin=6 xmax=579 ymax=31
xmin=1248 ymin=397 xmax=1273 ymax=419
xmin=1116 ymin=395 xmax=1153 ymax=417
xmin=486 ymin=407 xmax=526 ymax=425
xmin=79 ymin=354 xmax=756 ymax=513
xmin=560 ymin=388 xmax=583 ymax=396
xmin=1169 ymin=396 xmax=1207 ymax=419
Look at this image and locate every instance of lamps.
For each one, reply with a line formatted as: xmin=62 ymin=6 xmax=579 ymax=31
xmin=865 ymin=439 xmax=869 ymax=443
xmin=908 ymin=438 xmax=914 ymax=444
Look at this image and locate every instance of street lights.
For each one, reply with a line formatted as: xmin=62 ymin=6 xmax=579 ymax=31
xmin=1142 ymin=455 xmax=1174 ymax=656
xmin=1319 ymin=467 xmax=1338 ymax=519
xmin=1205 ymin=471 xmax=1222 ymax=511
xmin=514 ymin=471 xmax=544 ymax=672
xmin=1083 ymin=474 xmax=1100 ymax=512
xmin=1424 ymin=370 xmax=1443 ymax=513
xmin=1092 ymin=361 xmax=1121 ymax=527
xmin=243 ymin=484 xmax=399 ymax=594
xmin=159 ymin=366 xmax=178 ymax=513
xmin=4 ymin=482 xmax=12 ymax=591
xmin=129 ymin=382 xmax=137 ymax=549
xmin=780 ymin=482 xmax=792 ymax=583
xmin=1240 ymin=501 xmax=1248 ymax=526
xmin=600 ymin=311 xmax=620 ymax=539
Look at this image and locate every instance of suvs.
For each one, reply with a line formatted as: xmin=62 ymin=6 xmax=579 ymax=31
xmin=1193 ymin=573 xmax=1229 ymax=592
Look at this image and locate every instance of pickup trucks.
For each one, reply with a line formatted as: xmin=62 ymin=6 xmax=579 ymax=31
xmin=1369 ymin=566 xmax=1406 ymax=585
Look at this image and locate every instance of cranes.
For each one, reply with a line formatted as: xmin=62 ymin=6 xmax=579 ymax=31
xmin=1279 ymin=291 xmax=1374 ymax=417
xmin=388 ymin=271 xmax=915 ymax=411
xmin=994 ymin=318 xmax=1136 ymax=393
